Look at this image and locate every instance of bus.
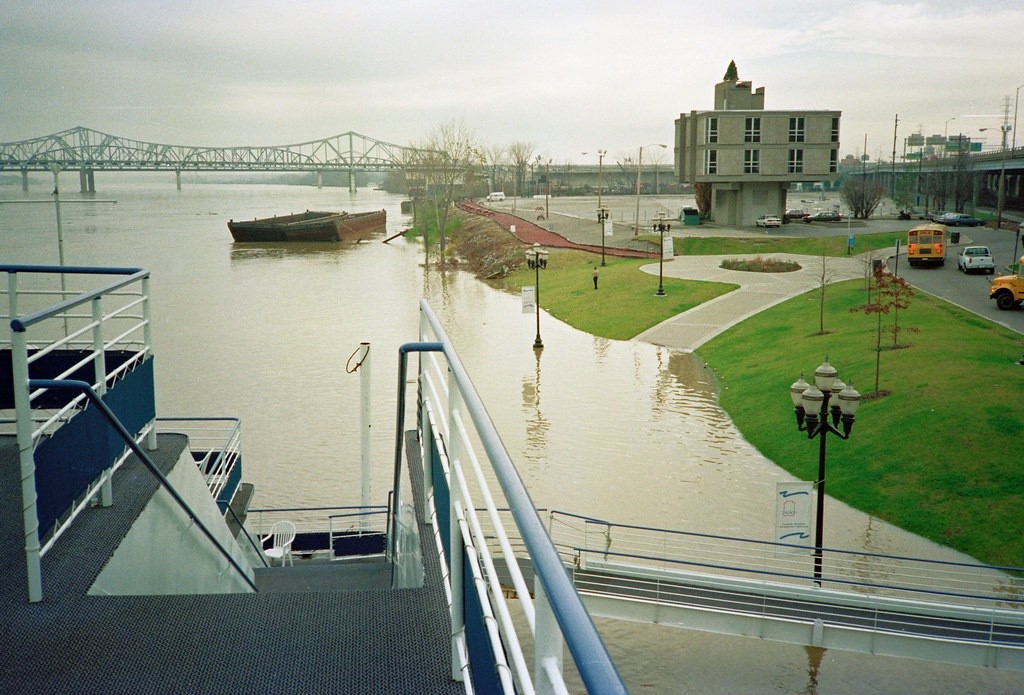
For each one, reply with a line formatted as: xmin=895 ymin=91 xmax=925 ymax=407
xmin=986 ymin=256 xmax=1024 ymax=311
xmin=907 ymin=224 xmax=947 ymax=265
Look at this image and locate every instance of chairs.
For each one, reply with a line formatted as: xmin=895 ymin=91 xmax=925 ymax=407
xmin=261 ymin=521 xmax=296 ymax=568
xmin=195 ymin=446 xmax=216 ymax=474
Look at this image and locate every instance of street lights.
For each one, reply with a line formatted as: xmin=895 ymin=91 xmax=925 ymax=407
xmin=652 ymin=212 xmax=671 ymax=296
xmin=596 ymin=204 xmax=610 ymax=266
xmin=635 ymin=144 xmax=667 ymax=235
xmin=582 ymin=152 xmax=602 ymax=222
xmin=526 ymin=242 xmax=549 ymax=348
xmin=945 ymin=117 xmax=956 ymax=136
xmin=979 ymin=127 xmax=1007 ymax=228
xmin=546 ymin=159 xmax=552 ymax=218
xmin=791 ymin=355 xmax=861 ymax=586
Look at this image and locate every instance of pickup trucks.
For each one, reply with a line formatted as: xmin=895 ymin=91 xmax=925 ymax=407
xmin=957 ymin=246 xmax=996 ymax=274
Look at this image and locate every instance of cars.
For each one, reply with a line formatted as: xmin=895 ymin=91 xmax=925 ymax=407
xmin=756 ymin=215 xmax=782 ymax=228
xmin=487 ymin=192 xmax=505 ymax=202
xmin=804 ymin=211 xmax=842 ymax=222
xmin=932 ymin=212 xmax=987 ymax=227
xmin=786 ymin=209 xmax=810 ymax=219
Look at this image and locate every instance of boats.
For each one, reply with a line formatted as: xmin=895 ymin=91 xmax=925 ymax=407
xmin=280 ymin=208 xmax=387 ymax=241
xmin=226 ymin=209 xmax=347 ymax=242
